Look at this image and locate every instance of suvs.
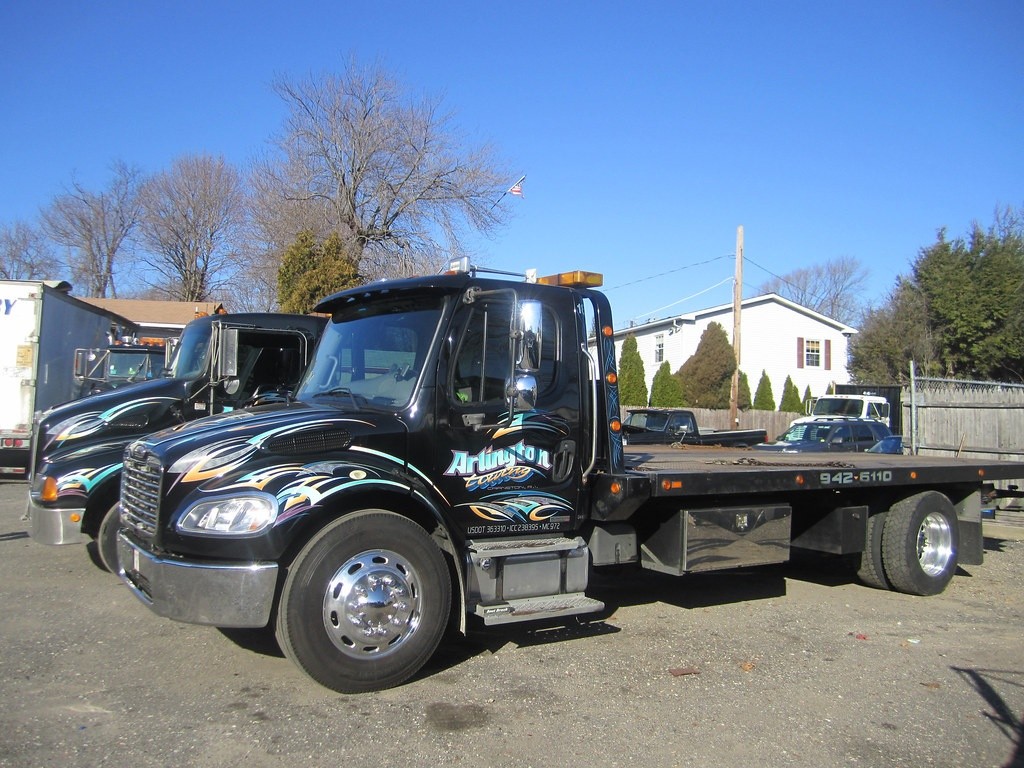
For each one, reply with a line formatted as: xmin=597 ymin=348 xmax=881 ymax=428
xmin=20 ymin=312 xmax=330 ymax=578
xmin=751 ymin=417 xmax=892 ymax=452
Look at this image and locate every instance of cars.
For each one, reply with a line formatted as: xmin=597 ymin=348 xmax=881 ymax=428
xmin=864 ymin=435 xmax=902 ymax=454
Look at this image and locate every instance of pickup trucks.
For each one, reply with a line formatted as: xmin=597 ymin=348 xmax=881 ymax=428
xmin=621 ymin=409 xmax=768 ymax=447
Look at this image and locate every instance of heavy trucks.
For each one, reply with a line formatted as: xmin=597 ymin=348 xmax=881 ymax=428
xmin=789 ymin=381 xmax=902 ymax=435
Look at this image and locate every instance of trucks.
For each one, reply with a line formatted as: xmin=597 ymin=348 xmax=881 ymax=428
xmin=0 ymin=281 xmax=140 ymax=474
xmin=116 ymin=255 xmax=1024 ymax=695
